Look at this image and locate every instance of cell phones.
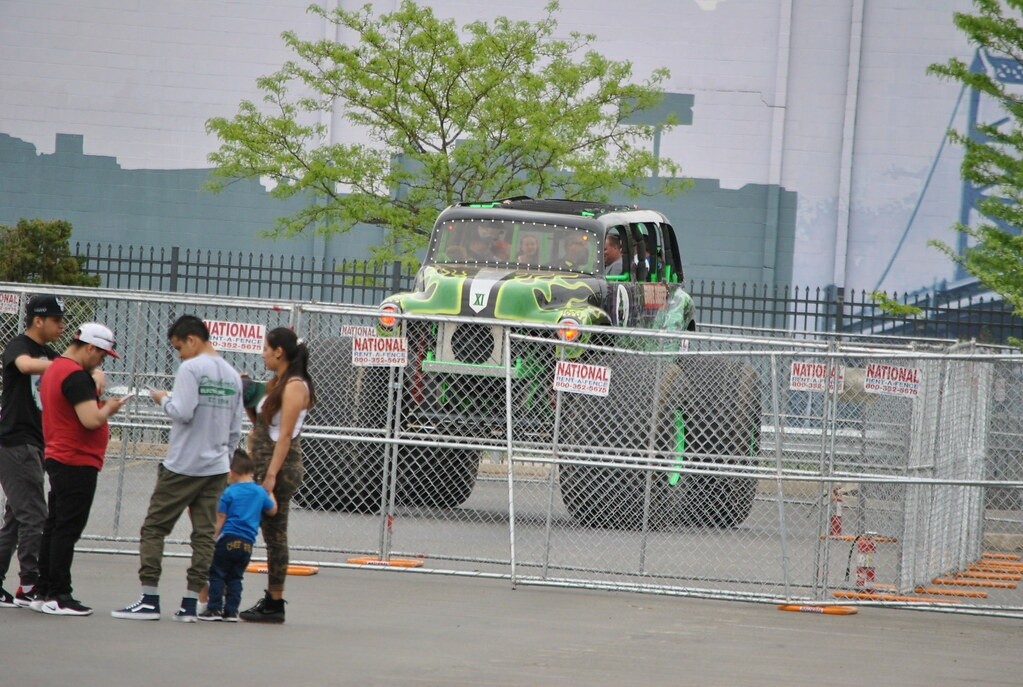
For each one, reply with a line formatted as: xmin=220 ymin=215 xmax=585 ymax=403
xmin=119 ymin=393 xmax=135 ymax=402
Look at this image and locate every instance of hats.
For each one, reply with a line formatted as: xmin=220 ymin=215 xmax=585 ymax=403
xmin=76 ymin=323 xmax=121 ymax=359
xmin=27 ymin=295 xmax=74 ymax=316
xmin=242 ymin=375 xmax=265 ymax=407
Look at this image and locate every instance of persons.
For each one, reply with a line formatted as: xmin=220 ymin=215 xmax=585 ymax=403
xmin=197 ymin=447 xmax=278 ymax=622
xmin=643 ymin=234 xmax=661 ymax=272
xmin=28 ymin=323 xmax=126 ymax=616
xmin=0 ymin=293 xmax=74 ymax=608
xmin=111 ymin=313 xmax=243 ymax=623
xmin=518 ymin=235 xmax=539 ymax=266
xmin=240 ymin=327 xmax=317 ymax=622
xmin=631 ymin=239 xmax=650 ymax=272
xmin=603 ymin=235 xmax=622 ymax=275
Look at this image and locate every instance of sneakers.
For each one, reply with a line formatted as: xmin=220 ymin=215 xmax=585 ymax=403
xmin=13 ymin=585 xmax=39 ymax=606
xmin=42 ymin=596 xmax=93 ymax=615
xmin=223 ymin=609 xmax=238 ymax=622
xmin=111 ymin=594 xmax=160 ymax=620
xmin=0 ymin=586 xmax=18 ymax=608
xmin=30 ymin=592 xmax=45 ymax=612
xmin=198 ymin=608 xmax=223 ymax=621
xmin=239 ymin=590 xmax=287 ymax=622
xmin=173 ymin=597 xmax=198 ymax=622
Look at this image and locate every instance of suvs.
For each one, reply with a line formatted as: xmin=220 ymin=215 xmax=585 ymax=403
xmin=259 ymin=195 xmax=765 ymax=532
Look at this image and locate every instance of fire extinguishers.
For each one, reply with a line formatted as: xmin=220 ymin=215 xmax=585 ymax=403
xmin=806 ymin=487 xmax=843 ymax=537
xmin=845 ymin=531 xmax=880 ymax=594
